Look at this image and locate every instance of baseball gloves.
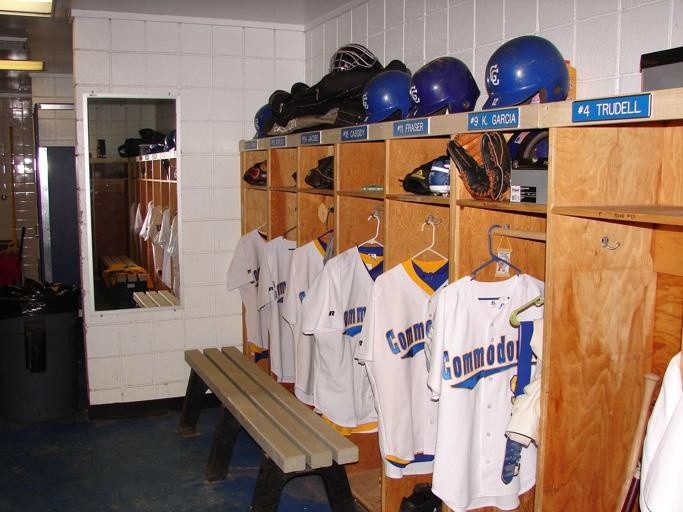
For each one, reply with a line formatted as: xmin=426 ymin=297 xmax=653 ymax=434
xmin=447 ymin=130 xmax=511 ymax=201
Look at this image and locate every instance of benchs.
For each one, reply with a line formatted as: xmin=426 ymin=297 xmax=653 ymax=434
xmin=132 ymin=289 xmax=178 ymax=308
xmin=102 ymin=254 xmax=148 ymax=282
xmin=176 ymin=345 xmax=358 ymax=512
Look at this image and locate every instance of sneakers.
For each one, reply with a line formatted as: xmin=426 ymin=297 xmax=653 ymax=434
xmin=430 ymin=160 xmax=450 ymax=195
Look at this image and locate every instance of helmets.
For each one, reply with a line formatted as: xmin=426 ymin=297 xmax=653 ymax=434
xmin=363 ymin=71 xmax=412 ymax=122
xmin=164 ymin=129 xmax=175 ymax=145
xmin=507 ymin=129 xmax=548 ymax=169
xmin=482 ymin=35 xmax=568 ymax=109
xmin=409 ymin=57 xmax=479 ymax=119
xmin=329 ymin=44 xmax=377 ymax=71
xmin=255 ymin=104 xmax=273 ymax=137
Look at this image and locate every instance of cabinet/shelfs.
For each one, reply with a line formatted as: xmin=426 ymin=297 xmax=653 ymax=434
xmin=241 ymin=88 xmax=683 ymax=510
xmin=88 ymin=151 xmax=179 ymax=289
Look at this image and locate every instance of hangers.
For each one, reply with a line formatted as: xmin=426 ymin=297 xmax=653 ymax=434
xmin=353 ymin=215 xmax=384 ymax=250
xmin=281 ymin=208 xmax=298 ymax=236
xmin=410 ymin=220 xmax=448 ymax=263
xmin=254 ymin=222 xmax=268 ymax=232
xmin=316 ymin=209 xmax=335 ymax=243
xmin=470 ymin=223 xmax=522 ymax=281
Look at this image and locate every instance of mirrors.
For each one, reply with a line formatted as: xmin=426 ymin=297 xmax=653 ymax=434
xmin=83 ymin=92 xmax=185 ymax=316
